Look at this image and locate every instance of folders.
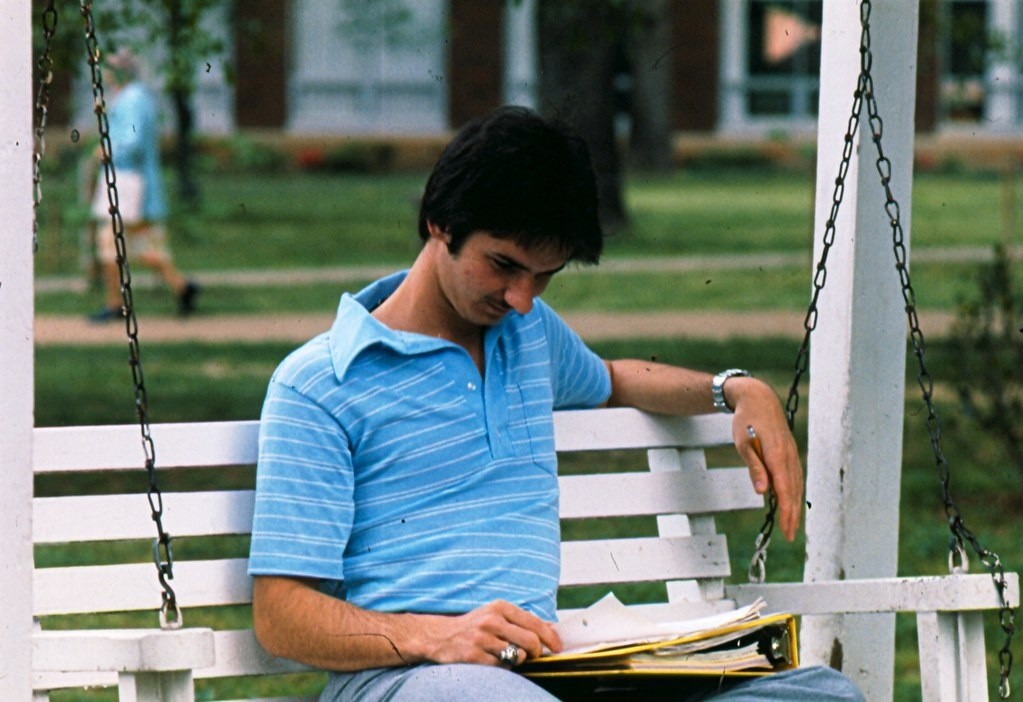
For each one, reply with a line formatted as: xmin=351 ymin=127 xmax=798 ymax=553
xmin=515 ymin=613 xmax=799 ymax=676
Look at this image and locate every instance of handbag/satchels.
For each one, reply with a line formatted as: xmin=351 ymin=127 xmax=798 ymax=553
xmin=90 ymin=165 xmax=145 ymax=226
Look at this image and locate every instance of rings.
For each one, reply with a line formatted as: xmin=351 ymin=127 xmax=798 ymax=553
xmin=500 ymin=644 xmax=518 ymax=666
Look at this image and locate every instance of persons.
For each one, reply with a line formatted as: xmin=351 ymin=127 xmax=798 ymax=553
xmin=86 ymin=43 xmax=199 ymax=320
xmin=248 ymin=105 xmax=867 ymax=702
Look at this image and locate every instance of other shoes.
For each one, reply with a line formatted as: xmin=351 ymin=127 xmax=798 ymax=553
xmin=88 ymin=306 xmax=125 ymax=322
xmin=178 ymin=282 xmax=197 ymax=315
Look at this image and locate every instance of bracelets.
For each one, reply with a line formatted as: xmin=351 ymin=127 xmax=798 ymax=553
xmin=712 ymin=368 xmax=752 ymax=414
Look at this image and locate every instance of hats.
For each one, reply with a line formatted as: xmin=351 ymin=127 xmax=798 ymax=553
xmin=104 ymin=45 xmax=142 ymax=80
